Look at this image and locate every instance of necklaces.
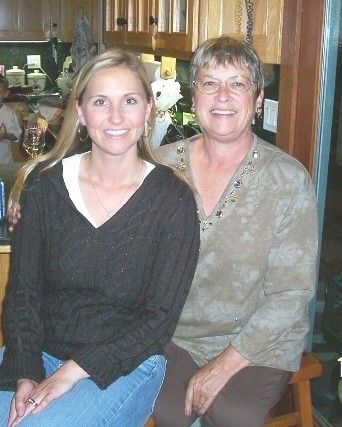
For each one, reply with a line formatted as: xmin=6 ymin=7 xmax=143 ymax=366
xmin=85 ymin=152 xmax=143 ymax=217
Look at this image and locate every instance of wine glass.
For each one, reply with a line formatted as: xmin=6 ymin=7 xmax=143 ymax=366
xmin=22 ymin=128 xmax=45 ymax=159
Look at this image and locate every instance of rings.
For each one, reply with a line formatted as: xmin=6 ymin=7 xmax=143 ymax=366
xmin=28 ymin=398 xmax=38 ymax=407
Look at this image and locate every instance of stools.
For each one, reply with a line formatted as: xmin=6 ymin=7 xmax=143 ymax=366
xmin=145 ymin=353 xmax=322 ymax=426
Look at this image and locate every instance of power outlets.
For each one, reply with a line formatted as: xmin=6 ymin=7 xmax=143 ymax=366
xmin=27 ymin=55 xmax=42 ymax=68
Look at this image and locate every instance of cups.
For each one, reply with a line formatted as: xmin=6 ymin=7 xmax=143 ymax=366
xmin=27 ymin=70 xmax=47 ymax=92
xmin=6 ymin=65 xmax=25 ymax=88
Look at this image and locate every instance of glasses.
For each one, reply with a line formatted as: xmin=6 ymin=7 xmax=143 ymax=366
xmin=193 ymin=75 xmax=259 ymax=94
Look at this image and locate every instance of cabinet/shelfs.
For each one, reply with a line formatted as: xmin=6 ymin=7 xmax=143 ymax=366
xmin=0 ymin=0 xmax=98 ymax=42
xmin=198 ymin=0 xmax=284 ymax=64
xmin=155 ymin=0 xmax=200 ymax=61
xmin=102 ymin=0 xmax=155 ymax=57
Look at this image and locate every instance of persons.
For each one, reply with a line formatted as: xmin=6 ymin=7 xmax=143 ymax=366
xmin=151 ymin=36 xmax=320 ymax=427
xmin=0 ymin=74 xmax=22 ymax=163
xmin=1 ymin=50 xmax=200 ymax=427
